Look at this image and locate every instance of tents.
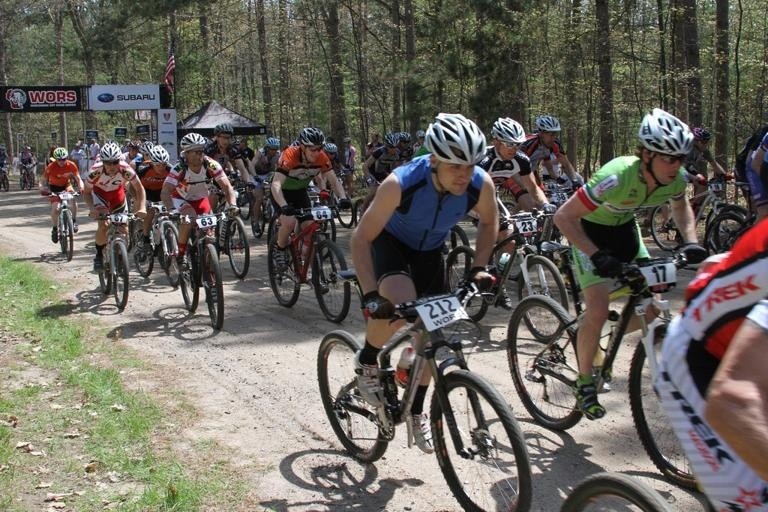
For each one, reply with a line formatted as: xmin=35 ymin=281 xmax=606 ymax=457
xmin=176 ymin=100 xmax=267 ymax=136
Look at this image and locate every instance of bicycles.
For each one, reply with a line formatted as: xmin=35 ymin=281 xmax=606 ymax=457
xmin=89 ymin=210 xmax=130 ymax=308
xmin=178 ymin=207 xmax=239 ymax=329
xmin=354 ymin=197 xmax=365 ymax=228
xmin=46 ymin=190 xmax=80 ymax=262
xmin=130 ymin=204 xmax=184 ymax=287
xmin=650 ymin=175 xmax=734 ymax=251
xmin=0 ymin=161 xmax=9 ymax=192
xmin=442 ymin=224 xmax=472 ymax=289
xmin=704 ymin=181 xmax=758 ymax=254
xmin=445 ymin=209 xmax=569 ymax=344
xmin=316 ymin=265 xmax=533 ymax=511
xmin=234 ymin=177 xmax=250 ymax=220
xmin=702 ymin=186 xmax=761 ymax=252
xmin=507 ymin=251 xmax=705 ymax=488
xmin=251 ymin=168 xmax=353 ymax=326
xmin=560 ymin=476 xmax=668 ymax=512
xmin=492 ymin=184 xmax=587 ymax=315
xmin=18 ymin=162 xmax=38 ymax=191
xmin=212 ymin=183 xmax=253 ymax=277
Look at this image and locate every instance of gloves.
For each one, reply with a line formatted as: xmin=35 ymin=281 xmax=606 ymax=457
xmin=245 ymin=182 xmax=256 ymax=190
xmin=590 ymin=250 xmax=621 ymax=278
xmin=254 ymin=175 xmax=263 ymax=183
xmin=167 ymin=209 xmax=181 ymax=222
xmin=338 ymin=198 xmax=351 ymax=209
xmin=227 ymin=206 xmax=239 ymax=216
xmin=696 ymin=174 xmax=709 ymax=185
xmin=721 ymin=172 xmax=738 ymax=182
xmin=469 ymin=267 xmax=494 ymax=291
xmin=555 ymin=176 xmax=566 ymax=185
xmin=361 ymin=291 xmax=396 ymax=320
xmin=281 ymin=206 xmax=294 ymax=215
xmin=572 ymin=180 xmax=582 ymax=192
xmin=542 ymin=203 xmax=558 ymax=216
xmin=680 ymin=243 xmax=708 ymax=263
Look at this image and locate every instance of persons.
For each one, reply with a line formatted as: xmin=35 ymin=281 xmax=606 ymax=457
xmin=644 ymin=202 xmax=671 ymax=238
xmin=503 ymin=117 xmax=581 ymax=240
xmin=249 ymin=137 xmax=282 ymax=233
xmin=543 ymin=149 xmax=567 ymax=185
xmin=129 ymin=146 xmax=174 ymax=248
xmin=323 ymin=129 xmax=425 ymax=216
xmin=746 ymin=131 xmax=768 ymax=224
xmin=39 ymin=147 xmax=85 ymax=243
xmin=82 ymin=144 xmax=146 ymax=267
xmin=468 ymin=118 xmax=558 ymax=313
xmin=70 ymin=139 xmax=153 ymax=171
xmin=553 ymin=109 xmax=708 ymax=421
xmin=242 ymin=136 xmax=255 ymax=160
xmin=649 ymin=216 xmax=768 ymax=511
xmin=45 ymin=145 xmax=57 ymax=164
xmin=204 ymin=124 xmax=256 ymax=208
xmin=160 ymin=133 xmax=241 ymax=285
xmin=351 ymin=114 xmax=500 ymax=452
xmin=671 ymin=127 xmax=730 ymax=245
xmin=230 ymin=135 xmax=255 ymax=179
xmin=269 ymin=127 xmax=351 ymax=292
xmin=540 ymin=148 xmax=560 ymax=183
xmin=0 ymin=144 xmax=39 ymax=183
xmin=734 ymin=125 xmax=768 ymax=221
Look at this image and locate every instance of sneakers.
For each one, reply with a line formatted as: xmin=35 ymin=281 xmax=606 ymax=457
xmin=142 ymin=242 xmax=151 ymax=255
xmin=93 ymin=255 xmax=103 ymax=271
xmin=499 ymin=288 xmax=513 ymax=310
xmin=254 ymin=222 xmax=260 ymax=233
xmin=52 ymin=230 xmax=58 ymax=243
xmin=73 ymin=220 xmax=78 ymax=232
xmin=593 ymin=365 xmax=612 ymax=382
xmin=206 ymin=282 xmax=217 ymax=303
xmin=412 ymin=414 xmax=434 ymax=454
xmin=273 ymin=250 xmax=288 ymax=272
xmin=178 ymin=255 xmax=189 ymax=271
xmin=355 ymin=350 xmax=384 ymax=407
xmin=572 ymin=380 xmax=605 ymax=419
xmin=318 ymin=279 xmax=329 ymax=294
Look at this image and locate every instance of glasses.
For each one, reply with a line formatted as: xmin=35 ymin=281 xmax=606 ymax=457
xmin=57 ymin=159 xmax=66 ymax=162
xmin=404 ymin=140 xmax=410 ymax=144
xmin=309 ymin=147 xmax=321 ymax=153
xmin=657 ymin=154 xmax=686 ymax=164
xmin=103 ymin=160 xmax=119 ymax=166
xmin=501 ymin=141 xmax=521 ymax=148
xmin=218 ymin=136 xmax=230 ymax=140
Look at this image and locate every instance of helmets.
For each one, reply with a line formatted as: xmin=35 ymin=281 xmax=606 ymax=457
xmin=537 ymin=115 xmax=561 ymax=132
xmin=638 ymin=107 xmax=694 ymax=157
xmin=492 ymin=117 xmax=526 ymax=144
xmin=128 ymin=141 xmax=138 ymax=149
xmin=149 ymin=145 xmax=169 ymax=165
xmin=53 ymin=148 xmax=69 ymax=159
xmin=416 ymin=130 xmax=426 ymax=139
xmin=180 ymin=133 xmax=206 ymax=151
xmin=290 ymin=141 xmax=301 ymax=147
xmin=384 ymin=134 xmax=398 ymax=147
xmin=325 ymin=143 xmax=338 ymax=153
xmin=344 ymin=138 xmax=351 ymax=143
xmin=214 ymin=124 xmax=233 ymax=135
xmin=266 ymin=138 xmax=280 ymax=149
xmin=140 ymin=141 xmax=154 ymax=155
xmin=299 ymin=128 xmax=325 ymax=146
xmin=692 ymin=127 xmax=712 ymax=141
xmin=424 ymin=113 xmax=486 ymax=166
xmin=100 ymin=142 xmax=123 ymax=161
xmin=400 ymin=132 xmax=412 ymax=141
